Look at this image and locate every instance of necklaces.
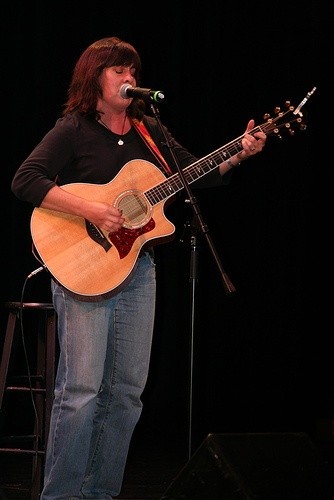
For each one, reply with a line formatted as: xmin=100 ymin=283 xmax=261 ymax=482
xmin=99 ymin=112 xmax=127 ymax=146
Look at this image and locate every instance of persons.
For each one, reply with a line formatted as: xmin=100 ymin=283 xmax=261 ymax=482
xmin=13 ymin=39 xmax=266 ymax=500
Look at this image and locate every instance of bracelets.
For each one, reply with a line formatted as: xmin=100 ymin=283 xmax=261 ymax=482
xmin=227 ymin=150 xmax=246 ymax=167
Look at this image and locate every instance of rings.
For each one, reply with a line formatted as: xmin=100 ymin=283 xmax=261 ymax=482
xmin=249 ymin=147 xmax=256 ymax=152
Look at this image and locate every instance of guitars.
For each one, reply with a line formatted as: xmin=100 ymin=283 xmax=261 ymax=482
xmin=29 ymin=85 xmax=318 ymax=302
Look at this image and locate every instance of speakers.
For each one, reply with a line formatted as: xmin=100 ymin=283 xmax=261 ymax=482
xmin=161 ymin=432 xmax=322 ymax=500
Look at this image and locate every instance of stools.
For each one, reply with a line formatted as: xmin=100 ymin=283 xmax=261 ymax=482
xmin=0 ymin=301 xmax=56 ymax=500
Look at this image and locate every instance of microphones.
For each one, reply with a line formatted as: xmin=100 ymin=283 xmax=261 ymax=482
xmin=119 ymin=83 xmax=165 ymax=102
